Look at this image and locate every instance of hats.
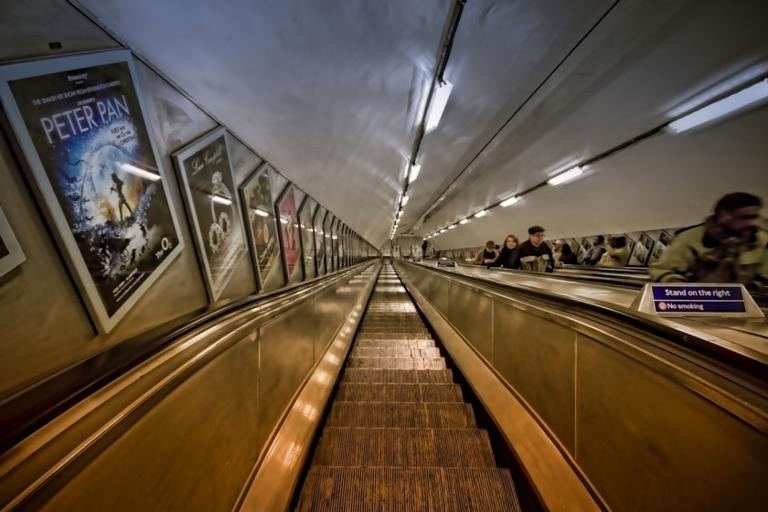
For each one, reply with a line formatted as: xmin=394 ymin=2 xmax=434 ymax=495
xmin=528 ymin=226 xmax=546 ymax=233
xmin=486 ymin=240 xmax=496 ymax=248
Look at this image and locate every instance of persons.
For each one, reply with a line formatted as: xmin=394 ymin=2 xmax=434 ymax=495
xmin=552 ymin=235 xmax=629 ymax=267
xmin=473 ymin=240 xmax=500 ymax=266
xmin=650 ymin=192 xmax=768 ymax=306
xmin=513 ymin=225 xmax=554 ymax=272
xmin=111 ymin=174 xmax=132 ymax=223
xmin=495 ymin=245 xmax=501 ymax=254
xmin=411 ymin=239 xmax=433 ymax=259
xmin=487 ymin=235 xmax=520 ymax=270
